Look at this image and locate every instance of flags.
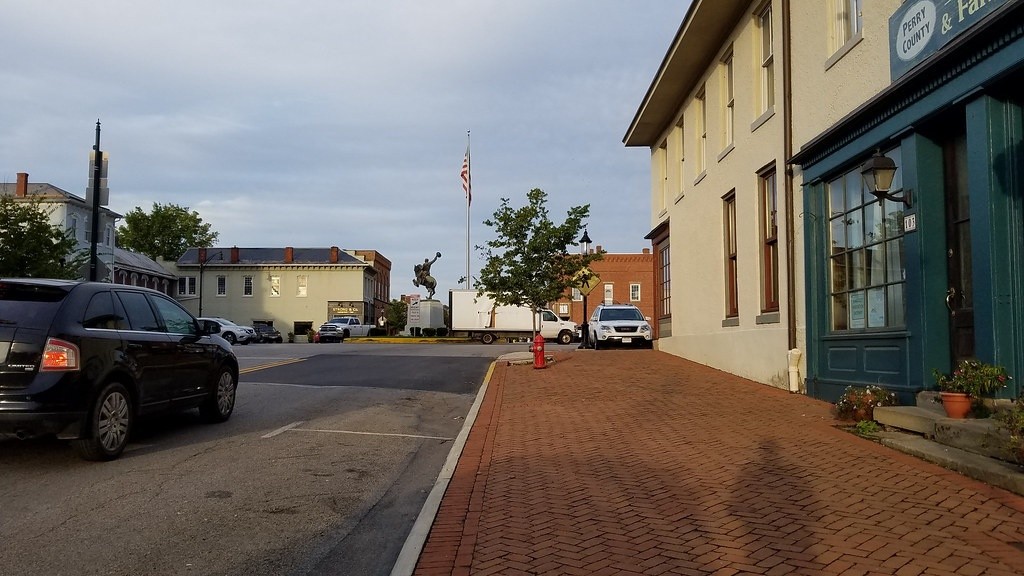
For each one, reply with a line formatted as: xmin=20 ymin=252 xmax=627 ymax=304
xmin=461 ymin=148 xmax=472 ymax=205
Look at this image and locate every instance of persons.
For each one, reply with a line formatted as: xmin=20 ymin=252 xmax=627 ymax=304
xmin=413 ymin=252 xmax=441 ymax=287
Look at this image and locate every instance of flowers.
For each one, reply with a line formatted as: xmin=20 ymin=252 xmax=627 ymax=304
xmin=930 ymin=357 xmax=1013 ymax=406
xmin=834 ymin=385 xmax=896 ymax=418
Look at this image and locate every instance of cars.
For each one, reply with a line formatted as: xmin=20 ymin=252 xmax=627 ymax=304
xmin=196 ymin=317 xmax=257 ymax=346
xmin=317 ymin=324 xmax=344 ymax=344
xmin=252 ymin=325 xmax=283 ymax=344
xmin=0 ymin=278 xmax=239 ymax=464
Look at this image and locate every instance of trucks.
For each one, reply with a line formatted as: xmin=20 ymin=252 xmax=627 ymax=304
xmin=449 ymin=288 xmax=578 ymax=345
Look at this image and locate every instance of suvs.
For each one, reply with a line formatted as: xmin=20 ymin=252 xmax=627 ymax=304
xmin=587 ymin=303 xmax=653 ymax=350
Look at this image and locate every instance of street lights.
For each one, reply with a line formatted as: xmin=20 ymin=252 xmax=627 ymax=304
xmin=381 ymin=310 xmax=384 ymax=322
xmin=199 ymin=251 xmax=224 ymax=319
xmin=578 ymin=229 xmax=592 ymax=350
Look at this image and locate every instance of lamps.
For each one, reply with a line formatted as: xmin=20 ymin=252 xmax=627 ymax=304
xmin=860 ymin=147 xmax=899 ymax=206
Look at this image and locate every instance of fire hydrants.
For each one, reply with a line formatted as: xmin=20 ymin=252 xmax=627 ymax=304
xmin=529 ymin=334 xmax=547 ymax=370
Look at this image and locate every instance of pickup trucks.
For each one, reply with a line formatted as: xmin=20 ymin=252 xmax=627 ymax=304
xmin=324 ymin=316 xmax=376 ymax=338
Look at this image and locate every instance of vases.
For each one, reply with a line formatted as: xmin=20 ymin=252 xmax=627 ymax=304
xmin=852 ymin=405 xmax=874 ymax=422
xmin=939 ymin=390 xmax=976 ymax=418
